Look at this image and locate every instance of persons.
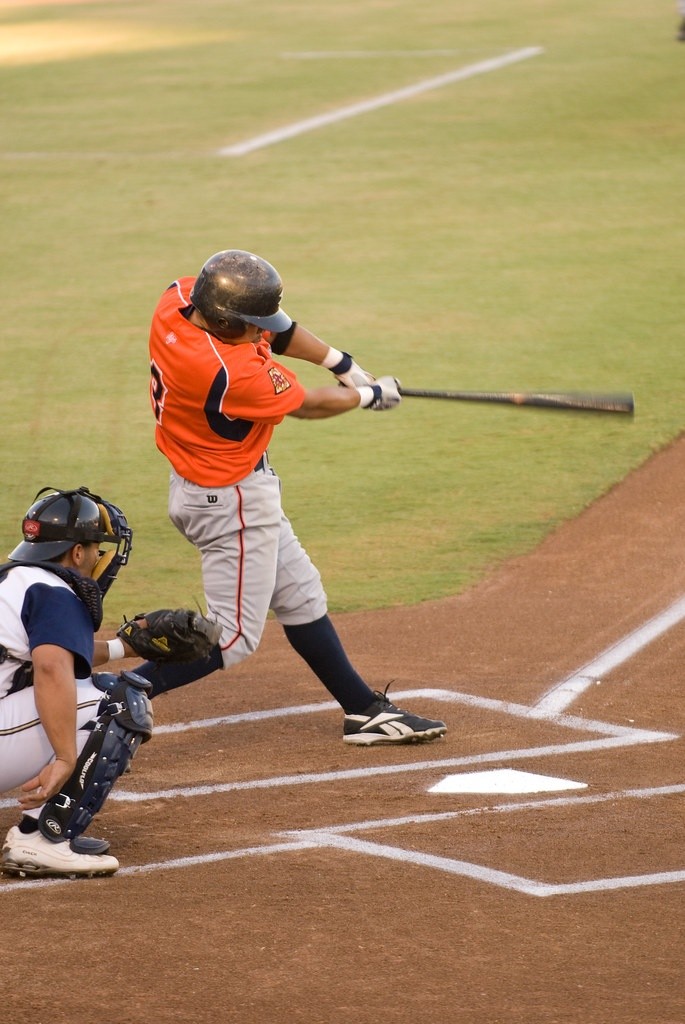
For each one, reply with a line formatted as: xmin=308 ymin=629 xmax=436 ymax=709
xmin=124 ymin=250 xmax=444 ymax=742
xmin=0 ymin=491 xmax=200 ymax=879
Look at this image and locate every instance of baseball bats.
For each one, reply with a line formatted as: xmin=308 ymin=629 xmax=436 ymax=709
xmin=396 ymin=388 xmax=637 ymax=416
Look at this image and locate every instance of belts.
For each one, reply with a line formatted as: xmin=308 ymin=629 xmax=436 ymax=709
xmin=254 ymin=453 xmax=269 ymax=472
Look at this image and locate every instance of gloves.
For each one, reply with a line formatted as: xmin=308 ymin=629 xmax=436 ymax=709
xmin=355 ymin=375 xmax=402 ymax=410
xmin=320 ymin=346 xmax=376 ymax=387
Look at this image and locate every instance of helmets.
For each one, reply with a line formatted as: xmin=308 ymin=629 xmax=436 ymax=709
xmin=189 ymin=250 xmax=292 ymax=339
xmin=7 ymin=492 xmax=108 ymax=563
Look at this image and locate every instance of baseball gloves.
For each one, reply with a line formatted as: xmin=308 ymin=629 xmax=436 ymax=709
xmin=117 ymin=606 xmax=224 ymax=666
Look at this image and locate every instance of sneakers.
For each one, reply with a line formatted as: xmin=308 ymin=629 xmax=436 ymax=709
xmin=0 ymin=825 xmax=119 ymax=880
xmin=343 ymin=678 xmax=447 ymax=746
